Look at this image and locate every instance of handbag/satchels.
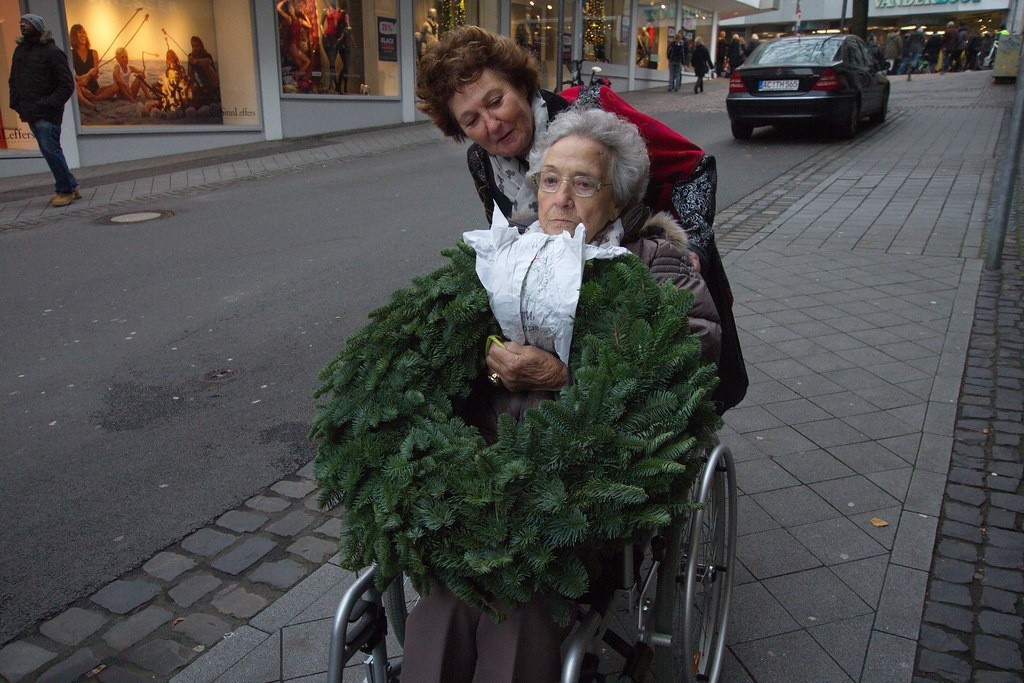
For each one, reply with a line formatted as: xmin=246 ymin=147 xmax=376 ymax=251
xmin=703 ymin=64 xmax=709 ymax=73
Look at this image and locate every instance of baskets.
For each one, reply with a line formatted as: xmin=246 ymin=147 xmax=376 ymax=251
xmin=566 ymin=60 xmax=578 ymax=73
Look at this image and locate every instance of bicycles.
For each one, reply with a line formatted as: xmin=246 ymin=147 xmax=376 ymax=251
xmin=554 ymin=58 xmax=612 ymax=98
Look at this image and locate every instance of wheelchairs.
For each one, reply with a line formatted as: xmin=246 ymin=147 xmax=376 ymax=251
xmin=325 ymin=433 xmax=736 ymax=683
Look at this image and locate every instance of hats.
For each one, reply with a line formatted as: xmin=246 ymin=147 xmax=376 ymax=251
xmin=20 ymin=15 xmax=44 ymax=33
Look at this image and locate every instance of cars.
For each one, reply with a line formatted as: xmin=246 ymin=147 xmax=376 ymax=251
xmin=726 ymin=33 xmax=891 ymax=142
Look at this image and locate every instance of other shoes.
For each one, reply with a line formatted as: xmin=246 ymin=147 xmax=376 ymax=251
xmin=52 ymin=186 xmax=80 ymax=205
xmin=668 ymin=86 xmax=673 ymax=92
xmin=674 ymin=86 xmax=680 ymax=92
xmin=700 ymin=89 xmax=704 ymax=93
xmin=694 ymin=87 xmax=698 ymax=94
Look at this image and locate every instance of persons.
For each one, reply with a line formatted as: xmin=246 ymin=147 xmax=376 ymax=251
xmin=69 ymin=25 xmax=119 ymax=112
xmin=691 ymin=36 xmax=714 ymax=94
xmin=666 ymin=30 xmax=689 ymax=91
xmin=320 ymin=0 xmax=357 ymax=94
xmin=400 ymin=111 xmax=722 ymax=683
xmin=422 ymin=8 xmax=439 ymax=38
xmin=637 ymin=21 xmax=654 ymax=64
xmin=9 ymin=14 xmax=81 ymax=207
xmin=897 ymin=23 xmax=925 ymax=74
xmin=276 ymin=0 xmax=312 ymax=79
xmin=113 ymin=47 xmax=152 ymax=102
xmin=728 ymin=33 xmax=760 ymax=73
xmin=868 ymin=22 xmax=1009 ymax=74
xmin=942 ymin=21 xmax=958 ymax=72
xmin=715 ymin=31 xmax=728 ymax=78
xmin=884 ymin=29 xmax=903 ymax=75
xmin=412 ymin=26 xmax=749 ymax=416
xmin=160 ymin=36 xmax=219 ymax=111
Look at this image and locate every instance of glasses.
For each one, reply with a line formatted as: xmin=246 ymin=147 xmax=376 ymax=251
xmin=530 ymin=172 xmax=614 ymax=198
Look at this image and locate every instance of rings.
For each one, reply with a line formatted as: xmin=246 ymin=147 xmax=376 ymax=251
xmin=488 ymin=373 xmax=499 ymax=383
